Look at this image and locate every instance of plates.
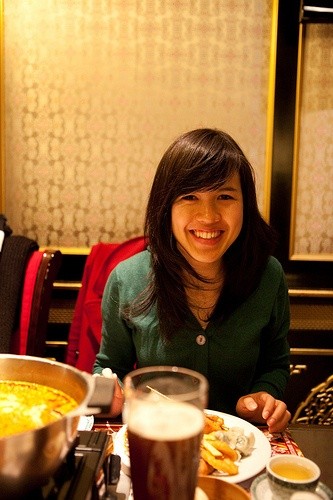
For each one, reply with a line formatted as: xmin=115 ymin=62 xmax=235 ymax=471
xmin=250 ymin=472 xmax=333 ymax=500
xmin=112 ymin=408 xmax=272 ymax=485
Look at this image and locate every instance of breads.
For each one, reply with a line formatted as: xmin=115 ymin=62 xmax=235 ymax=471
xmin=199 ymin=459 xmax=216 ymax=477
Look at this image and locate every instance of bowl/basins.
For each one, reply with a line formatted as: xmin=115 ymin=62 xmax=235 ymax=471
xmin=194 ymin=476 xmax=252 ymax=500
xmin=0 ymin=353 xmax=96 ymax=498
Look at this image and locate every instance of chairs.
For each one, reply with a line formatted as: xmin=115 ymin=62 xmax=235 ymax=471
xmin=16 ymin=236 xmax=150 ymax=373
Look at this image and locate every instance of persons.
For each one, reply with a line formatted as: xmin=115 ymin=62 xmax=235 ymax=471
xmin=92 ymin=129 xmax=291 ymax=433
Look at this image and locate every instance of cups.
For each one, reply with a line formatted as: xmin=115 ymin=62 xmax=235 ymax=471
xmin=123 ymin=366 xmax=208 ymax=500
xmin=266 ymin=454 xmax=321 ymax=500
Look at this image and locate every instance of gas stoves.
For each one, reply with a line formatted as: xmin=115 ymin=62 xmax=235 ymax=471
xmin=0 ymin=430 xmax=121 ymax=500
